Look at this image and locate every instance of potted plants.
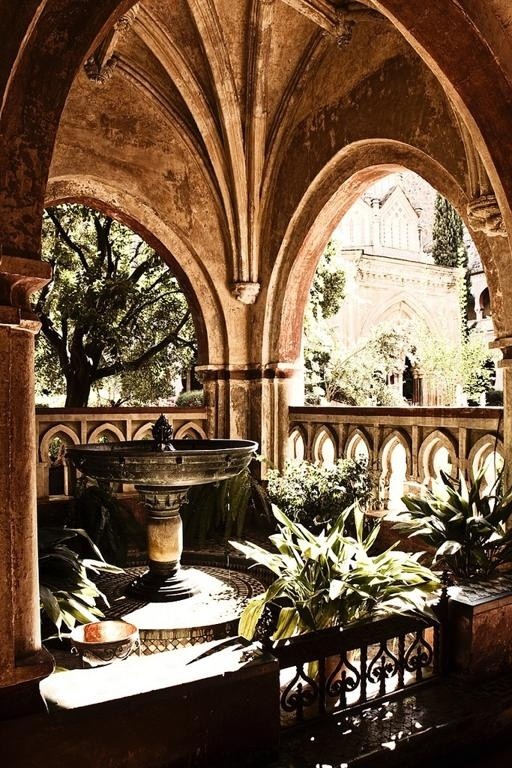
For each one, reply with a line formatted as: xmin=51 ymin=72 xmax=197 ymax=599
xmin=226 ymin=494 xmax=442 ymax=684
